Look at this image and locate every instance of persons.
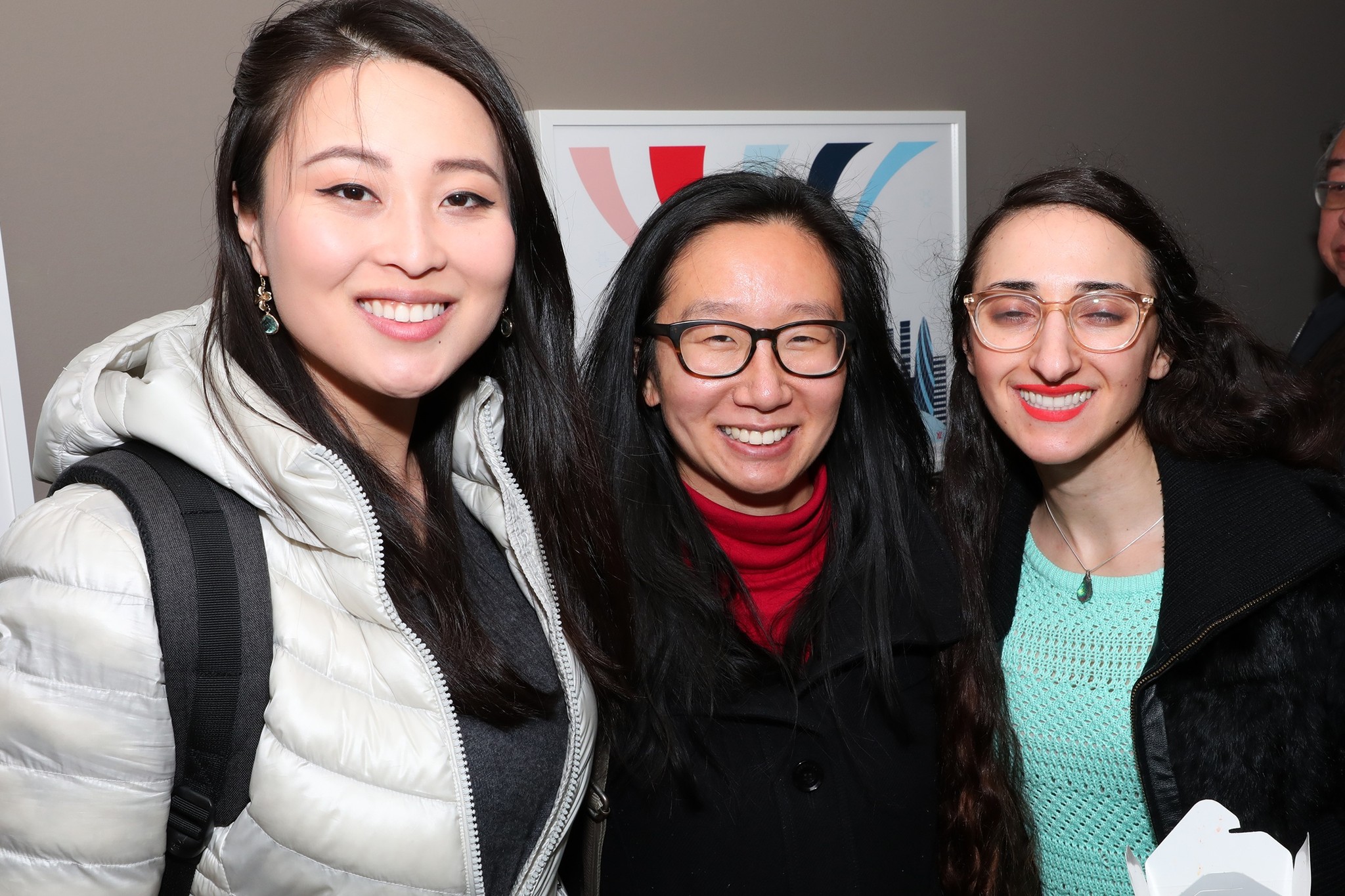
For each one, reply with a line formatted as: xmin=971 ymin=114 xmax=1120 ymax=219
xmin=575 ymin=170 xmax=974 ymax=896
xmin=940 ymin=168 xmax=1345 ymax=896
xmin=1 ymin=0 xmax=634 ymax=895
xmin=1286 ymin=111 xmax=1343 ymax=428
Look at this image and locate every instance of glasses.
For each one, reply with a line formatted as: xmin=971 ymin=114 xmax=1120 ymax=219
xmin=1314 ymin=182 xmax=1345 ymax=210
xmin=642 ymin=318 xmax=855 ymax=380
xmin=963 ymin=289 xmax=1158 ymax=353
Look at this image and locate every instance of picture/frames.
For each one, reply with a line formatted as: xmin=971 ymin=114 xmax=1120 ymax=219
xmin=522 ymin=109 xmax=972 ymax=477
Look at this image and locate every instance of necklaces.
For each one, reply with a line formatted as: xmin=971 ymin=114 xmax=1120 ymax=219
xmin=1043 ymin=497 xmax=1161 ymax=602
